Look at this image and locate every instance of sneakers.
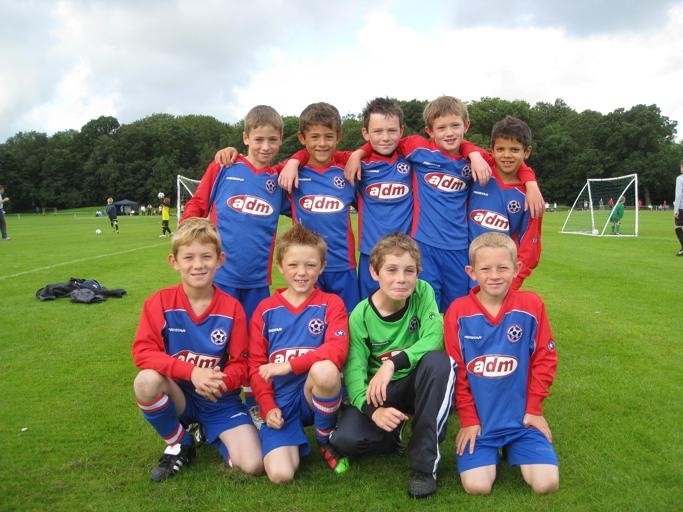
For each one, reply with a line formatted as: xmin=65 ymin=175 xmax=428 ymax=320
xmin=190 ymin=425 xmax=204 ymax=447
xmin=150 ymin=434 xmax=197 ymax=482
xmin=407 ymin=471 xmax=437 ymax=498
xmin=676 ymin=250 xmax=683 ymax=256
xmin=317 ymin=441 xmax=350 ymax=475
xmin=159 ymin=235 xmax=167 ymax=239
xmin=388 ymin=420 xmax=407 ymax=446
xmin=168 ymin=232 xmax=174 ymax=237
xmin=248 ymin=406 xmax=264 ymax=430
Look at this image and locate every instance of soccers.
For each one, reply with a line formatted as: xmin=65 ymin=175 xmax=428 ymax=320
xmin=266 ymin=179 xmax=276 ymax=192
xmin=211 ymin=328 xmax=227 ymax=346
xmin=409 ymin=317 xmax=419 ymax=332
xmin=508 ymin=200 xmax=521 ymax=214
xmin=333 ymin=176 xmax=345 ymax=188
xmin=461 ymin=165 xmax=472 ymax=177
xmin=96 ymin=229 xmax=102 ymax=235
xmin=157 ymin=192 xmax=165 ymax=200
xmin=396 ymin=162 xmax=410 ymax=174
xmin=308 ymin=317 xmax=324 ymax=335
xmin=508 ymin=325 xmax=522 ymax=343
xmin=592 ymin=228 xmax=600 ymax=237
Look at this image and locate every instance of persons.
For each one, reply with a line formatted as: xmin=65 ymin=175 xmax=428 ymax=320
xmin=140 ymin=204 xmax=145 ymax=216
xmin=608 ymin=196 xmax=626 ymax=234
xmin=443 ymin=232 xmax=559 ymax=495
xmin=0 ymin=185 xmax=12 ymax=241
xmin=598 ymin=198 xmax=604 ymax=209
xmin=130 ymin=209 xmax=136 ymax=214
xmin=157 ymin=197 xmax=174 ymax=240
xmin=178 ymin=105 xmax=294 ymax=328
xmin=544 ymin=199 xmax=551 ymax=212
xmin=40 ymin=199 xmax=47 ymax=216
xmin=465 ymin=117 xmax=541 ymax=307
xmin=100 ymin=198 xmax=120 ymax=233
xmin=249 ymin=222 xmax=349 ymax=485
xmin=673 ymin=159 xmax=683 ymax=256
xmin=553 ymin=200 xmax=557 ymax=213
xmin=95 ymin=210 xmax=102 ymax=216
xmin=583 ymin=199 xmax=589 ymax=210
xmin=329 ymin=232 xmax=457 ymax=498
xmin=133 ymin=217 xmax=265 ymax=485
xmin=278 ymin=96 xmax=492 ymax=302
xmin=213 ymin=101 xmax=361 ymax=317
xmin=158 ymin=204 xmax=162 ymax=215
xmin=147 ymin=202 xmax=152 ymax=215
xmin=343 ymin=97 xmax=545 ymax=314
